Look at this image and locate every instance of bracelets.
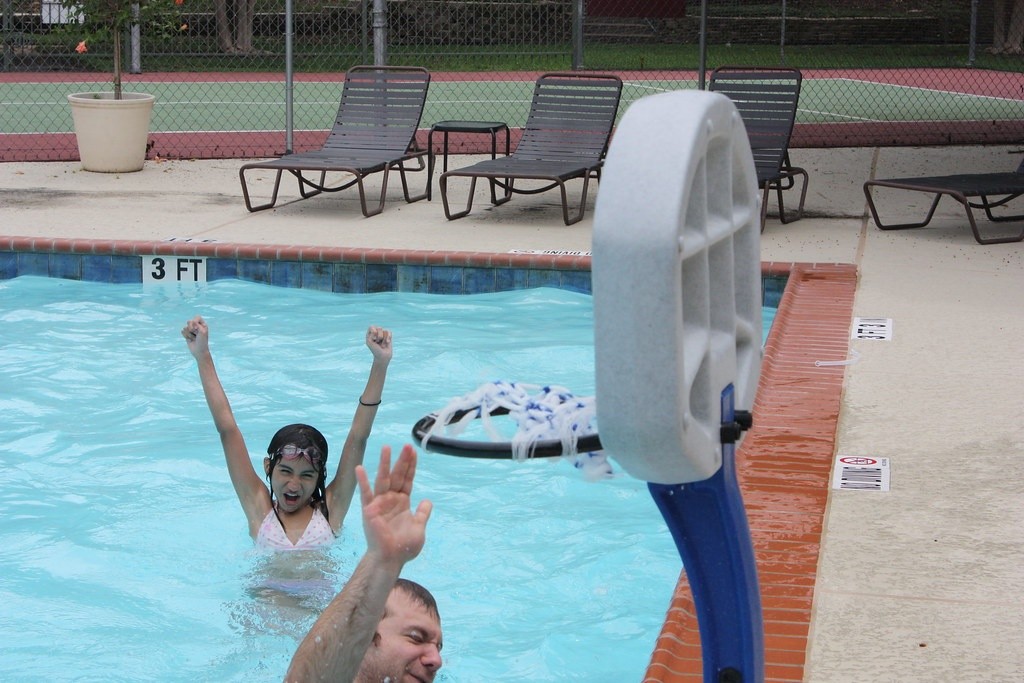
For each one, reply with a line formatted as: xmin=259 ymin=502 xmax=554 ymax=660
xmin=359 ymin=396 xmax=381 ymax=406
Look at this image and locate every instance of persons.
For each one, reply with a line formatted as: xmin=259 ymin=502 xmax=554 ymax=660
xmin=283 ymin=442 xmax=443 ymax=683
xmin=182 ymin=315 xmax=392 ymax=549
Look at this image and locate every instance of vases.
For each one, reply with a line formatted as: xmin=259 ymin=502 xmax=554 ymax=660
xmin=67 ymin=93 xmax=154 ymax=173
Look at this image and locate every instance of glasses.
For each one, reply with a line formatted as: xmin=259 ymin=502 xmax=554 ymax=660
xmin=272 ymin=444 xmax=321 ymax=464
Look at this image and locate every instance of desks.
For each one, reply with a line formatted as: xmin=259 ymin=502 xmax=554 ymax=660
xmin=426 ymin=120 xmax=511 ymax=202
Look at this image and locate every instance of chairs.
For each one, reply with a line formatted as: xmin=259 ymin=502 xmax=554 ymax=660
xmin=240 ymin=65 xmax=436 ymax=217
xmin=709 ymin=65 xmax=809 ymax=232
xmin=863 ymin=150 xmax=1024 ymax=244
xmin=440 ymin=73 xmax=623 ymax=225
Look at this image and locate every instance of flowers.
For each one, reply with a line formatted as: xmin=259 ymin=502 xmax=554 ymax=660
xmin=60 ymin=0 xmax=187 ymax=99
